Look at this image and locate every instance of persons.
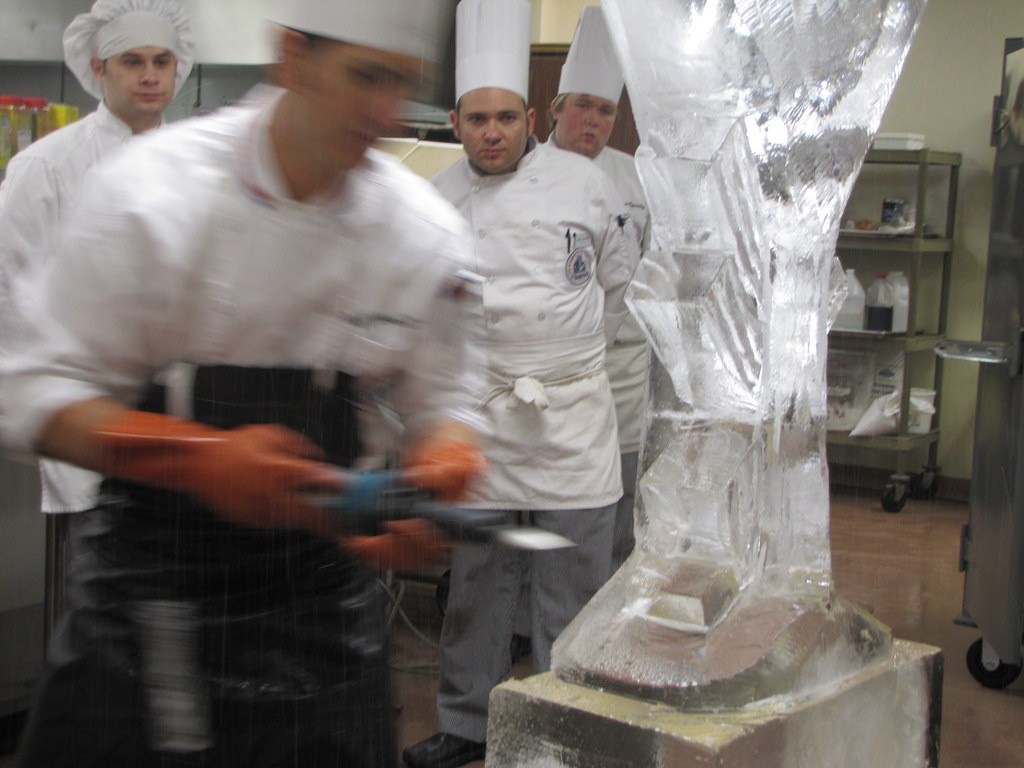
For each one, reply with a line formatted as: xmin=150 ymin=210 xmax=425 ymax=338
xmin=0 ymin=1 xmax=197 ymax=553
xmin=512 ymin=5 xmax=654 ymax=664
xmin=2 ymin=1 xmax=485 ymax=766
xmin=404 ymin=0 xmax=639 ymax=768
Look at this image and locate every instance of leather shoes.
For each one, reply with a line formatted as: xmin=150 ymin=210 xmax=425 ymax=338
xmin=402 ymin=732 xmax=486 ymax=767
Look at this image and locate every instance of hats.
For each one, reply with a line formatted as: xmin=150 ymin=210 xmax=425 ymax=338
xmin=62 ymin=0 xmax=199 ymax=103
xmin=268 ymin=0 xmax=442 ymax=64
xmin=455 ymin=0 xmax=532 ymax=112
xmin=557 ymin=5 xmax=625 ymax=106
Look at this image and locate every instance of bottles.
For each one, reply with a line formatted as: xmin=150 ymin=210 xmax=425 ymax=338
xmin=835 ymin=269 xmax=908 ymax=334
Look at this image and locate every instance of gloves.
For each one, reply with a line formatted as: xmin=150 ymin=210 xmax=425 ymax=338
xmin=89 ymin=408 xmax=491 ymax=572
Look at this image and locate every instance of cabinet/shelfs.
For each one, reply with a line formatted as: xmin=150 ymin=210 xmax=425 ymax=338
xmin=827 ymin=147 xmax=962 ymax=514
